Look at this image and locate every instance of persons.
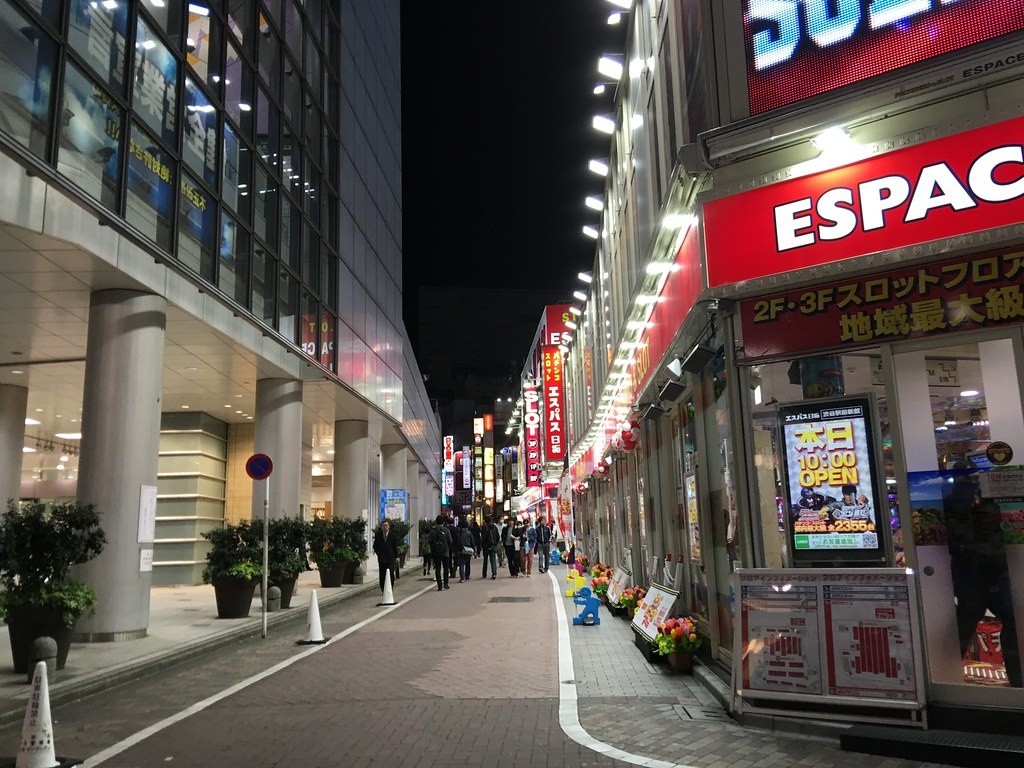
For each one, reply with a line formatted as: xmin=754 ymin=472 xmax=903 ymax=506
xmin=372 ymin=521 xmax=399 ymax=597
xmin=940 ymin=460 xmax=978 ymax=506
xmin=422 ymin=514 xmax=552 ymax=591
xmin=823 ymin=484 xmax=872 ymax=523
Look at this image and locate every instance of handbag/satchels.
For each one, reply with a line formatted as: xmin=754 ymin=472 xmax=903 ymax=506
xmin=462 ymin=546 xmax=474 ymax=556
xmin=514 ymin=540 xmax=520 ymax=551
xmin=525 ymin=541 xmax=530 ymax=555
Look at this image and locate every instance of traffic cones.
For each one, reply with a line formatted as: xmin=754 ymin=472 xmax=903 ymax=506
xmin=375 ymin=568 xmax=399 ymax=606
xmin=10 ymin=661 xmax=84 ymax=768
xmin=294 ymin=589 xmax=332 ymax=644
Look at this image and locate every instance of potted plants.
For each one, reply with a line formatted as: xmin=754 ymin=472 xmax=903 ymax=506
xmin=419 ymin=518 xmax=433 ymax=557
xmin=199 ymin=506 xmax=368 ymax=618
xmin=0 ymin=495 xmax=111 ymax=675
xmin=370 ymin=517 xmax=414 ymax=567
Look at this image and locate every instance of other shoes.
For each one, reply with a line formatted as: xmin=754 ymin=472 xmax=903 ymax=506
xmin=522 ymin=569 xmax=526 ymax=575
xmin=423 ymin=569 xmax=426 ymax=576
xmin=466 ymin=575 xmax=469 ymax=580
xmin=511 ymin=573 xmax=519 ymax=578
xmin=483 ymin=574 xmax=487 ymax=579
xmin=450 ymin=572 xmax=455 ymax=577
xmin=438 ymin=588 xmax=442 ymax=591
xmin=539 ymin=568 xmax=545 ymax=573
xmin=461 ymin=578 xmax=464 ymax=582
xmin=527 ymin=570 xmax=531 ymax=577
xmin=428 ymin=571 xmax=431 ymax=574
xmin=444 ymin=585 xmax=450 ymax=588
xmin=545 ymin=568 xmax=548 ymax=573
xmin=492 ymin=574 xmax=496 ymax=579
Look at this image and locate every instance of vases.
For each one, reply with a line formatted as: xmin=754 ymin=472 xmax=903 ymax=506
xmin=597 ymin=593 xmax=608 ymax=604
xmin=665 ymin=652 xmax=693 ymax=675
xmin=625 ymin=606 xmax=636 ymax=618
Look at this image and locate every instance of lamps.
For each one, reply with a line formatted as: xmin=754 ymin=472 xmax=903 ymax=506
xmin=35 ymin=438 xmax=79 ymax=456
xmin=592 ymin=111 xmax=617 ymax=134
xmin=557 ymin=157 xmax=609 ymax=352
xmin=750 ymin=375 xmax=762 ymax=390
xmin=679 ymin=344 xmax=718 ymax=375
xmin=94 ymin=147 xmax=115 ymax=164
xmin=597 ymin=53 xmax=624 ymax=81
xmin=811 ymin=127 xmax=852 ymax=149
xmin=62 ymin=108 xmax=76 ymax=129
xmin=504 ymin=377 xmax=543 ymax=434
xmin=604 ymin=456 xmax=616 ymax=466
xmin=640 ymin=404 xmax=666 ymax=423
xmin=593 ymin=80 xmax=618 ymax=94
xmin=659 ymin=377 xmax=687 ymax=402
xmin=607 ymin=9 xmax=632 ymax=25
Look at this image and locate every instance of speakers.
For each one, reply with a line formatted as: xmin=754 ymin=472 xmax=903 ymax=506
xmin=659 ymin=380 xmax=686 ymax=403
xmin=643 ymin=404 xmax=664 ymax=422
xmin=681 ymin=344 xmax=716 ymax=374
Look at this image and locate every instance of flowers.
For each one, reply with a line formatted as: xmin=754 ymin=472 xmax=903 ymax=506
xmin=573 ymin=554 xmax=704 ymax=657
